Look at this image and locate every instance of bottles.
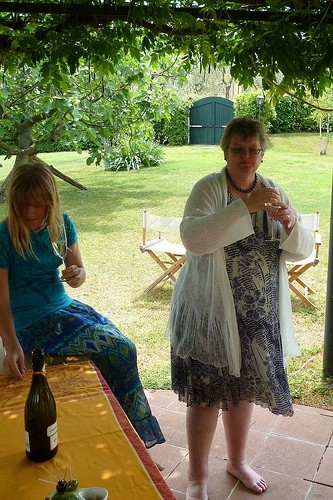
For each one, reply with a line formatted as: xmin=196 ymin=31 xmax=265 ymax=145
xmin=25 ymin=348 xmax=59 ymax=463
xmin=0 ymin=336 xmax=6 ymax=373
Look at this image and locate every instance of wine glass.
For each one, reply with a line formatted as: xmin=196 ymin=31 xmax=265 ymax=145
xmin=46 ymin=223 xmax=74 ymax=282
xmin=265 ymin=186 xmax=282 ymax=241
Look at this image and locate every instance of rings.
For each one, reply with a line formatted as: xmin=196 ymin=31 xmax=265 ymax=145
xmin=287 ymin=218 xmax=291 ymax=222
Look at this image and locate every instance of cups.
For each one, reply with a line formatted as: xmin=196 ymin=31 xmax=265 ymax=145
xmin=75 ymin=487 xmax=109 ymax=500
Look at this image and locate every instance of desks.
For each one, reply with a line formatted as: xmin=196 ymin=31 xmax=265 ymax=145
xmin=0 ymin=355 xmax=177 ymax=500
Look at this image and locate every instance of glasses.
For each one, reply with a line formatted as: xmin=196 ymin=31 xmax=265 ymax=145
xmin=226 ymin=145 xmax=263 ymax=155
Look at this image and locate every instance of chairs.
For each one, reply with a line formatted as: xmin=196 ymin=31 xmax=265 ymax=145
xmin=286 ymin=211 xmax=321 ymax=308
xmin=139 ymin=210 xmax=188 ymax=294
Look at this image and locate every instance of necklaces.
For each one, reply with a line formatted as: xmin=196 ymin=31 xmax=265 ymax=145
xmin=226 ymin=169 xmax=258 ymax=192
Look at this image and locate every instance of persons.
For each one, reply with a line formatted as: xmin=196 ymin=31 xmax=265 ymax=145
xmin=0 ymin=162 xmax=165 ymax=474
xmin=168 ymin=116 xmax=311 ymax=500
